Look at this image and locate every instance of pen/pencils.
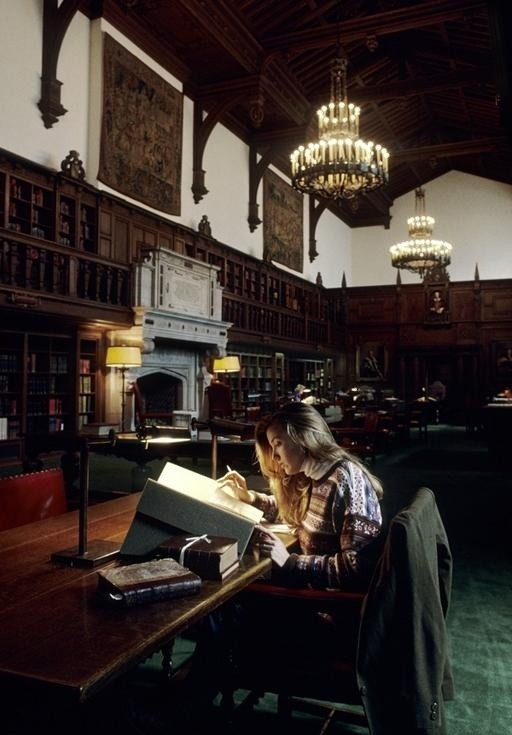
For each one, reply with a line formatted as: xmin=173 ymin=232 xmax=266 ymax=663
xmin=225 ymin=464 xmax=241 ymax=490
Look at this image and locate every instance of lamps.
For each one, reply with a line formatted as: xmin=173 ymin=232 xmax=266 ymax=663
xmin=105 ymin=343 xmax=144 ymax=433
xmin=213 ymin=356 xmax=243 ymax=375
xmin=49 ymin=416 xmax=256 ymax=567
xmin=289 ymin=55 xmax=392 ymax=211
xmin=389 ymin=185 xmax=453 ymax=278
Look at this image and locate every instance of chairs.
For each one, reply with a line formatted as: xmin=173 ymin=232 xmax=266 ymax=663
xmin=243 ymin=487 xmax=444 ymax=735
xmin=208 ymin=382 xmax=248 ymax=424
xmin=329 ymin=399 xmax=429 ymax=467
xmin=1 ymin=468 xmax=68 ymax=530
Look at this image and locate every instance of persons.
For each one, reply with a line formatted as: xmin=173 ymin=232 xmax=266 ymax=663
xmin=180 ymin=400 xmax=383 ymax=643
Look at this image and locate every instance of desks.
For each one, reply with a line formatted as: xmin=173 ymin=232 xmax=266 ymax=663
xmin=0 ymin=491 xmax=299 ymax=735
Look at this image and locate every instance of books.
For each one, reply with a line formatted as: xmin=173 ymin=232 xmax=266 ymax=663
xmin=96 ymin=557 xmax=206 ymax=606
xmin=2 ymin=177 xmax=340 ymax=442
xmin=119 ymin=459 xmax=270 ymax=565
xmin=158 ymin=531 xmax=241 ymax=581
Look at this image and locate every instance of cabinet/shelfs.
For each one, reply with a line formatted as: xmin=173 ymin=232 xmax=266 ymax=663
xmin=222 ymin=353 xmax=324 ymax=415
xmin=184 ymin=225 xmax=315 ymax=318
xmin=1 ymin=148 xmax=97 ymax=268
